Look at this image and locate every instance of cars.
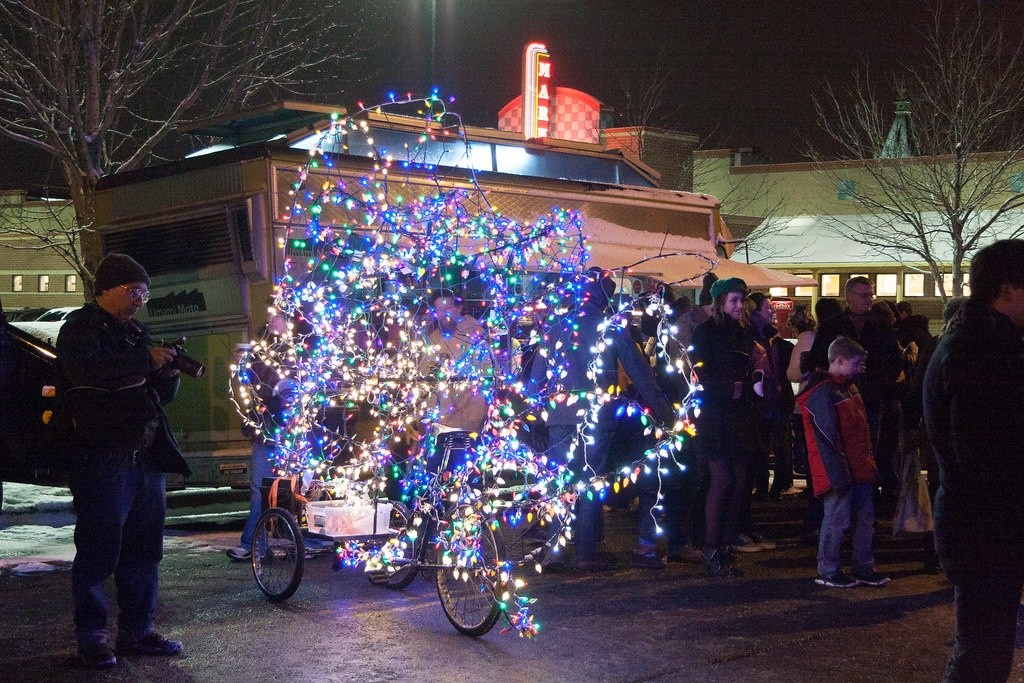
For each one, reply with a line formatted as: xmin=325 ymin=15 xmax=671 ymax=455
xmin=2 ymin=306 xmax=83 ymax=327
xmin=0 ymin=303 xmax=60 ymax=482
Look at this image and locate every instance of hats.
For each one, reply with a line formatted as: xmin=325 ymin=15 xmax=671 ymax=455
xmin=710 ymin=278 xmax=747 ymax=300
xmin=92 ymin=252 xmax=151 ymax=297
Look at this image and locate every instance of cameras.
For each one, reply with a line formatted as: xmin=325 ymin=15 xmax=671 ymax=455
xmin=163 ymin=336 xmax=205 ymax=378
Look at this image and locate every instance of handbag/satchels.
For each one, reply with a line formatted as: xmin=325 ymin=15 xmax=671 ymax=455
xmin=892 ymin=454 xmax=933 ymax=537
xmin=59 ymin=374 xmax=158 ymax=446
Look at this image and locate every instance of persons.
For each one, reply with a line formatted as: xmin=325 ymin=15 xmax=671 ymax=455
xmin=579 ymin=475 xmax=588 ymax=497
xmin=527 ymin=267 xmax=675 ymax=572
xmin=226 ymin=301 xmax=315 ymax=561
xmin=406 ymin=287 xmax=492 ymax=435
xmin=921 ymin=240 xmax=1024 ymax=683
xmin=50 ymin=254 xmax=194 ymax=668
xmin=795 ymin=336 xmax=892 ymax=589
xmin=689 ymin=277 xmax=756 ymax=579
xmin=618 ymin=276 xmax=971 ymax=569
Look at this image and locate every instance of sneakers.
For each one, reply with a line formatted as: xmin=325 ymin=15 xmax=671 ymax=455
xmin=82 ymin=644 xmax=119 ymax=670
xmin=814 ymin=570 xmax=859 ymax=587
xmin=227 ymin=545 xmax=252 ymax=560
xmin=115 ymin=629 xmax=183 ymax=655
xmin=272 ymin=545 xmax=288 ymax=561
xmin=850 ymin=568 xmax=892 ymax=587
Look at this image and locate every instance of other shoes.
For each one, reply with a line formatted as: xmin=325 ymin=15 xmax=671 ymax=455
xmin=576 ymin=556 xmax=618 ymax=572
xmin=563 ymin=530 xmax=605 ymax=546
xmin=662 ymin=537 xmax=703 ymax=562
xmin=533 ymin=556 xmax=566 ymax=574
xmin=730 ymin=533 xmax=762 ymax=554
xmin=633 ymin=545 xmax=668 ymax=569
xmin=702 ymin=545 xmax=744 ymax=578
xmin=521 ymin=525 xmax=547 ymax=543
xmin=751 ymin=529 xmax=777 ymax=549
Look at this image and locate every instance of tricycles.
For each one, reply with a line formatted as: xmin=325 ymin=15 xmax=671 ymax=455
xmin=249 ymin=392 xmax=510 ymax=639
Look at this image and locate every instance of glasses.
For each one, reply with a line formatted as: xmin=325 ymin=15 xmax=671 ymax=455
xmin=120 ymin=284 xmax=150 ymax=305
xmin=852 ymin=290 xmax=876 ymax=303
